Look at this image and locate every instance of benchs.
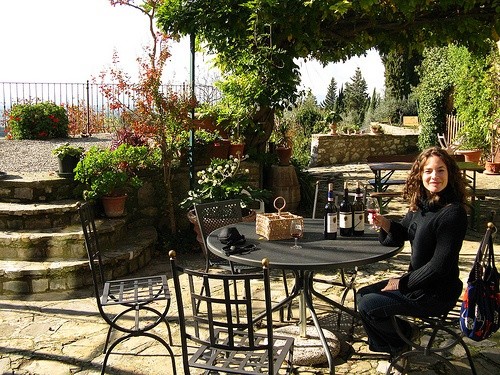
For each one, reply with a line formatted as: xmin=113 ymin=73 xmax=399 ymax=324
xmin=370 ymin=189 xmax=500 ymax=232
xmin=369 ymin=179 xmax=472 ymax=192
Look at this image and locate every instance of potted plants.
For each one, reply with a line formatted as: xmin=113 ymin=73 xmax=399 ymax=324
xmin=324 ymin=112 xmax=343 ymax=136
xmin=455 ymin=123 xmax=500 ymax=175
xmin=53 ymin=127 xmax=295 ymax=217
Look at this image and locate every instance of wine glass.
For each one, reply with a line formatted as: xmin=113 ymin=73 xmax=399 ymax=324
xmin=367 ymin=198 xmax=380 ymax=233
xmin=290 ymin=220 xmax=302 ymax=250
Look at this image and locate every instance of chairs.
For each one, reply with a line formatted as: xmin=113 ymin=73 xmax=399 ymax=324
xmin=76 ymin=192 xmax=176 ymax=375
xmin=195 ymin=199 xmax=292 ymax=326
xmin=286 ymin=181 xmax=368 ymax=330
xmin=169 ymin=250 xmax=294 ymax=375
xmin=386 ymin=209 xmax=497 ymax=375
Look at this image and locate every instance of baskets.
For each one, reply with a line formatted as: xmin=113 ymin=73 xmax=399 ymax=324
xmin=255 ymin=211 xmax=304 ymax=240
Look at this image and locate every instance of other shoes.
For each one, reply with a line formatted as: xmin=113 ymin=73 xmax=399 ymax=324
xmin=391 ymin=344 xmax=412 ymax=375
xmin=411 ymin=324 xmax=423 ymax=342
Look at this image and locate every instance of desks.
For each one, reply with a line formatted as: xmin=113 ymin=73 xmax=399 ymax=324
xmin=206 ymin=218 xmax=404 ymax=375
xmin=369 ymin=162 xmax=486 ymax=229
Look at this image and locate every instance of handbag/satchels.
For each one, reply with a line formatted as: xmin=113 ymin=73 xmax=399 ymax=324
xmin=459 ymin=236 xmax=499 ymax=342
xmin=218 ymin=225 xmax=257 ymax=256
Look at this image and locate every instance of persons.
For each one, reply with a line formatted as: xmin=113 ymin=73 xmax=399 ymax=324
xmin=355 ymin=146 xmax=466 ymax=356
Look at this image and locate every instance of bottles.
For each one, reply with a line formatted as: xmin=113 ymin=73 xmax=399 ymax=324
xmin=339 ymin=182 xmax=353 ymax=237
xmin=323 ymin=183 xmax=338 ymax=240
xmin=352 ymin=182 xmax=365 ymax=237
